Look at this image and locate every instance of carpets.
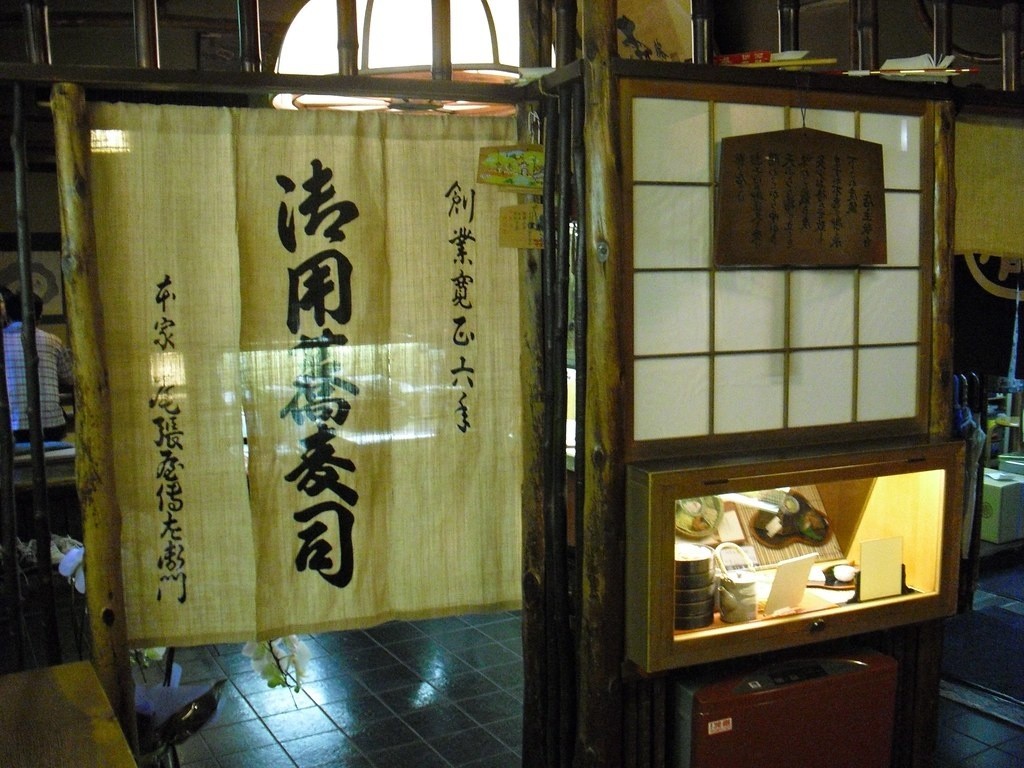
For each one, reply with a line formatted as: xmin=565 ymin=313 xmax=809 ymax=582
xmin=937 ymin=542 xmax=1023 ymax=705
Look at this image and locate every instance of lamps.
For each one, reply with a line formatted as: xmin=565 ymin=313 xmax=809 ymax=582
xmin=269 ymin=1 xmax=556 ymax=115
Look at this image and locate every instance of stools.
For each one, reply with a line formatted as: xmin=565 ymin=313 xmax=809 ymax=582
xmin=987 ymin=416 xmax=1022 ymax=466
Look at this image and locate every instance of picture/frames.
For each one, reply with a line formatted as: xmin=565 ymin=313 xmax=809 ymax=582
xmin=0 ymin=232 xmax=68 ymax=323
xmin=155 ymin=13 xmax=274 ymax=72
xmin=0 ymin=15 xmax=139 ymax=169
xmin=199 ymin=30 xmax=240 ymax=74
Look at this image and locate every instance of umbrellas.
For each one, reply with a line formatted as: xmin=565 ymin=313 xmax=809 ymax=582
xmin=959 ymin=374 xmax=978 ymax=521
xmin=953 ymin=375 xmax=965 ymax=440
xmin=961 ymin=371 xmax=989 ymax=562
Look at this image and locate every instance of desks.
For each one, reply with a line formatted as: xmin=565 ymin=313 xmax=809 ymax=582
xmin=1 ymin=661 xmax=137 ymax=768
xmin=9 ymin=454 xmax=80 ymax=617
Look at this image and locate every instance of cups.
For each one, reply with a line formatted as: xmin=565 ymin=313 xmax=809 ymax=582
xmin=833 ymin=565 xmax=856 ymax=580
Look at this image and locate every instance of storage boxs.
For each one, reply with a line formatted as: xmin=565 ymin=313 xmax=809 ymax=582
xmin=980 ymin=468 xmax=1018 ymax=545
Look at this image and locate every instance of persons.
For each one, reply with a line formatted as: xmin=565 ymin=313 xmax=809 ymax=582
xmin=1 ymin=291 xmax=79 ymax=444
xmin=1 ymin=285 xmax=16 ymax=325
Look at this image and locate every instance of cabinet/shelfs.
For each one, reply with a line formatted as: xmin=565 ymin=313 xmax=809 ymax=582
xmin=625 ymin=434 xmax=959 ymax=672
xmin=669 ymin=643 xmax=898 ymax=766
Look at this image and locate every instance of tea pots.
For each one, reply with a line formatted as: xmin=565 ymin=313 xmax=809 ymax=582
xmin=714 ymin=542 xmax=758 ymax=623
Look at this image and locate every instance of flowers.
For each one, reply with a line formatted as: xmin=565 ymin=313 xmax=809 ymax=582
xmin=243 ymin=636 xmax=311 ymax=693
